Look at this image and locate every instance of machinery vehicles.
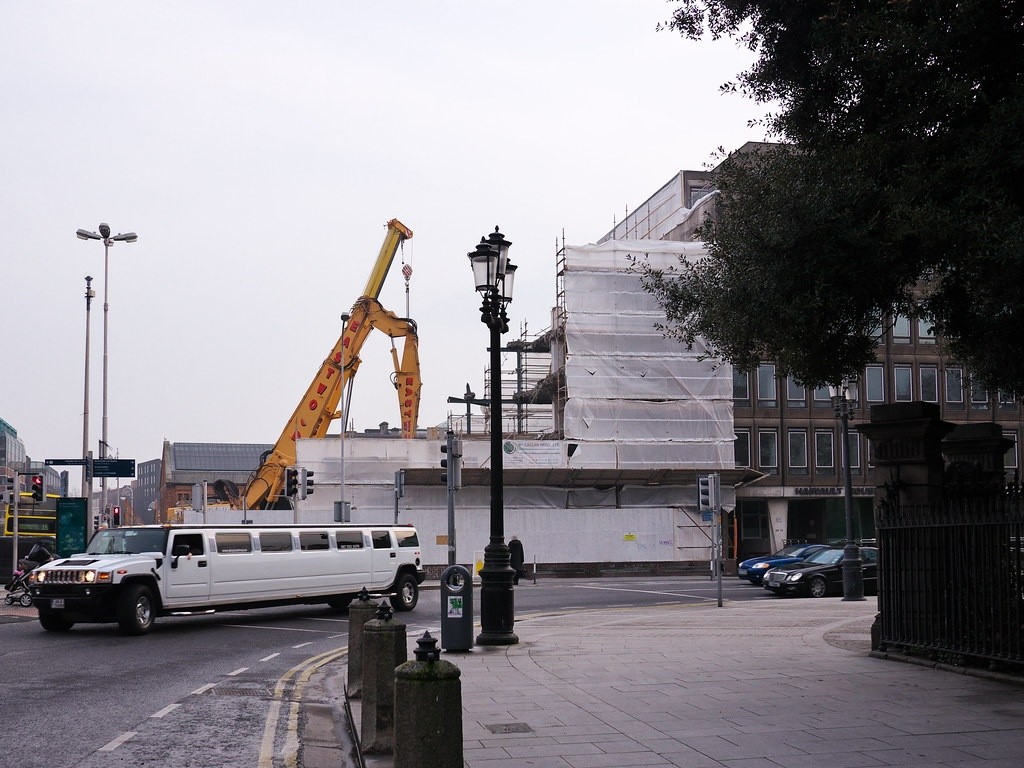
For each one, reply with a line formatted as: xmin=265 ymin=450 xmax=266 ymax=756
xmin=160 ymin=216 xmax=420 ymax=526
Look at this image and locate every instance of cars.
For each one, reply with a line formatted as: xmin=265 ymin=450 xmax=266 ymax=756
xmin=736 ymin=543 xmax=832 ymax=587
xmin=31 ymin=521 xmax=428 ymax=636
xmin=762 ymin=545 xmax=879 ymax=598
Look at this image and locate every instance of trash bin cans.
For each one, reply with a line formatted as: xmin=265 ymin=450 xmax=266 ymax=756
xmin=439 ymin=564 xmax=475 ymax=653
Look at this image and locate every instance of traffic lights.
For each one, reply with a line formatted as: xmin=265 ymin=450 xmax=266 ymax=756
xmin=0 ymin=489 xmax=9 ymax=503
xmin=31 ymin=474 xmax=47 ymax=503
xmin=113 ymin=505 xmax=121 ymax=525
xmin=698 ymin=476 xmax=714 ymax=510
xmin=93 ymin=515 xmax=100 ymax=530
xmin=300 ymin=467 xmax=315 ymax=500
xmin=6 ymin=476 xmax=15 ymax=501
xmin=440 ymin=442 xmax=447 ymax=481
xmin=285 ymin=467 xmax=298 ymax=501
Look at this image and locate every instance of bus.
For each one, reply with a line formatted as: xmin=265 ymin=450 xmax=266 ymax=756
xmin=3 ymin=491 xmax=63 ymax=537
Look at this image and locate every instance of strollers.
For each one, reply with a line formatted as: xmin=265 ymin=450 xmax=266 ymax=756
xmin=4 ymin=558 xmax=54 ymax=608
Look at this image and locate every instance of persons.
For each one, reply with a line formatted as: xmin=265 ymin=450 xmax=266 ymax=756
xmin=508 ymin=534 xmax=524 ymax=585
xmin=187 ymin=545 xmax=194 ymax=560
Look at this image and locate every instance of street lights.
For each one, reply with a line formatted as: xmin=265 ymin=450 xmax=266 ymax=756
xmin=467 ymin=225 xmax=520 ymax=645
xmin=828 ymin=362 xmax=867 ymax=602
xmin=75 ymin=219 xmax=140 ymax=524
